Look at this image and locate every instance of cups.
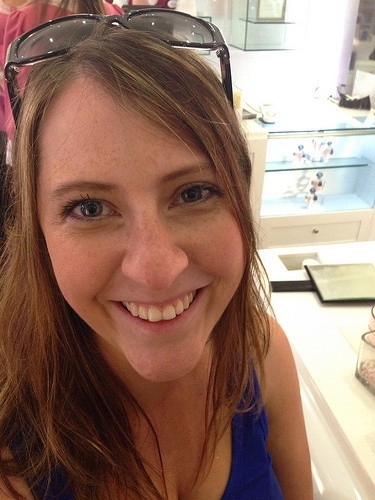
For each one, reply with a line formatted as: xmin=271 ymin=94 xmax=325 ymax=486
xmin=355 ymin=331 xmax=375 ymax=395
xmin=260 ymin=103 xmax=278 ymax=123
xmin=368 ymin=305 xmax=375 ymax=331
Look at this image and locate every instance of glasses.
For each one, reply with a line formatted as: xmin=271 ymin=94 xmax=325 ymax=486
xmin=4 ymin=8 xmax=233 ymax=129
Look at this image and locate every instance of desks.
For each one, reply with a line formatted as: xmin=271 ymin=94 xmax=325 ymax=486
xmin=251 ymin=241 xmax=374 ymax=499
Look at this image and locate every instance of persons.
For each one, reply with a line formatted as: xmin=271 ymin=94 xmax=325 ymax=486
xmin=0 ymin=8 xmax=315 ymax=500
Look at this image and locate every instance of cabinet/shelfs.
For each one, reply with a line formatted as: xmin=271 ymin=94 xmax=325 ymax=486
xmin=239 ymin=99 xmax=375 ymax=247
